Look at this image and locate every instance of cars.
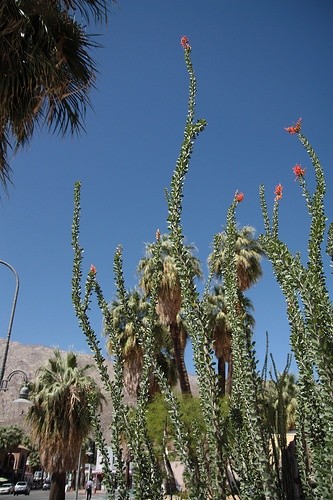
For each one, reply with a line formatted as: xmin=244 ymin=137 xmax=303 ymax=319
xmin=14 ymin=481 xmax=31 ymax=495
xmin=0 ymin=482 xmax=14 ymax=495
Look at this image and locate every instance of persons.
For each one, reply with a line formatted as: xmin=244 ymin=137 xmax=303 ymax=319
xmin=85 ymin=476 xmax=94 ymax=500
